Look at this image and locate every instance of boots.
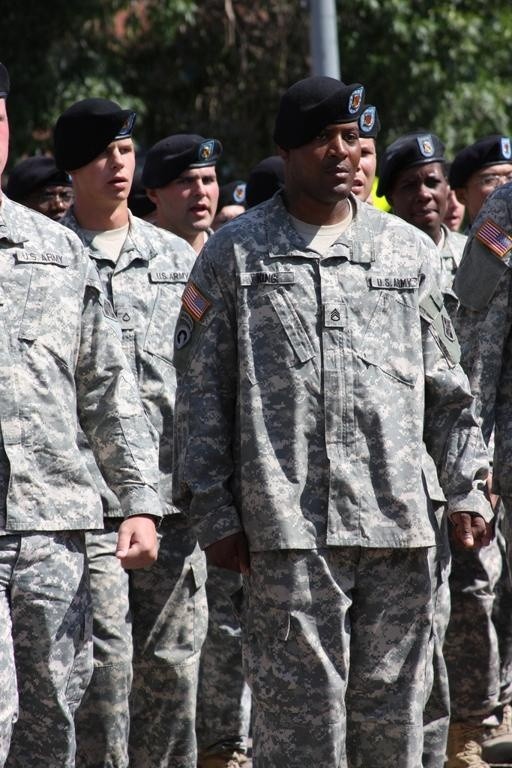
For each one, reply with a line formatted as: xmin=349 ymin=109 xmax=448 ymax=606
xmin=445 ymin=705 xmax=512 ymax=768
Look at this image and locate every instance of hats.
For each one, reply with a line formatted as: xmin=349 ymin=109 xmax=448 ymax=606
xmin=143 ymin=135 xmax=223 ymax=189
xmin=449 ymin=137 xmax=511 ymax=190
xmin=247 ymin=155 xmax=285 ymax=208
xmin=2 ymin=155 xmax=73 ymax=200
xmin=50 ymin=99 xmax=135 ymax=170
xmin=275 ymin=75 xmax=364 ymax=148
xmin=359 ymin=103 xmax=380 ymax=138
xmin=216 ymin=179 xmax=244 ymax=216
xmin=377 ymin=133 xmax=445 ymax=198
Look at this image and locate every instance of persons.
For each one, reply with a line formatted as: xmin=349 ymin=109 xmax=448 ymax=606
xmin=0 ymin=75 xmax=512 ymax=767
